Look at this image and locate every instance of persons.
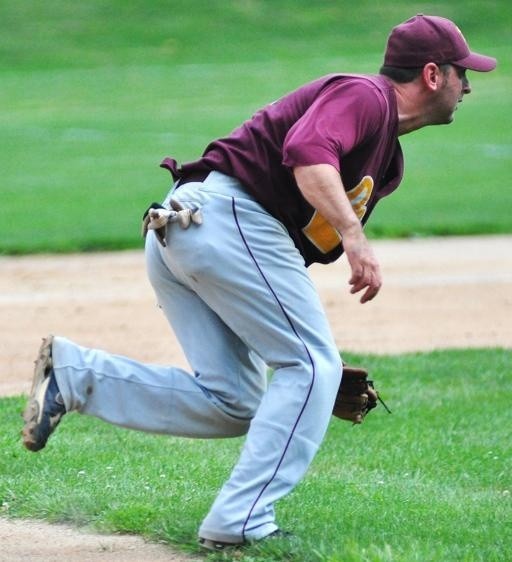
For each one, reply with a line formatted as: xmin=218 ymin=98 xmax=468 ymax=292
xmin=22 ymin=13 xmax=497 ymax=551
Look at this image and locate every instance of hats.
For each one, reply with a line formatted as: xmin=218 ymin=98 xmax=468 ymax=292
xmin=384 ymin=14 xmax=497 ymax=72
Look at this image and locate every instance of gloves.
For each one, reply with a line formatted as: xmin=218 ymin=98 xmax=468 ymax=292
xmin=141 ymin=198 xmax=202 ymax=247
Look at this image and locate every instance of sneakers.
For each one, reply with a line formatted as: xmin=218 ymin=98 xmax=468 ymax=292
xmin=199 ymin=529 xmax=295 ymax=551
xmin=21 ymin=334 xmax=65 ymax=452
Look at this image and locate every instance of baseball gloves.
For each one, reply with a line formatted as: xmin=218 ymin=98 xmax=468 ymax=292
xmin=336 ymin=364 xmax=379 ymax=424
